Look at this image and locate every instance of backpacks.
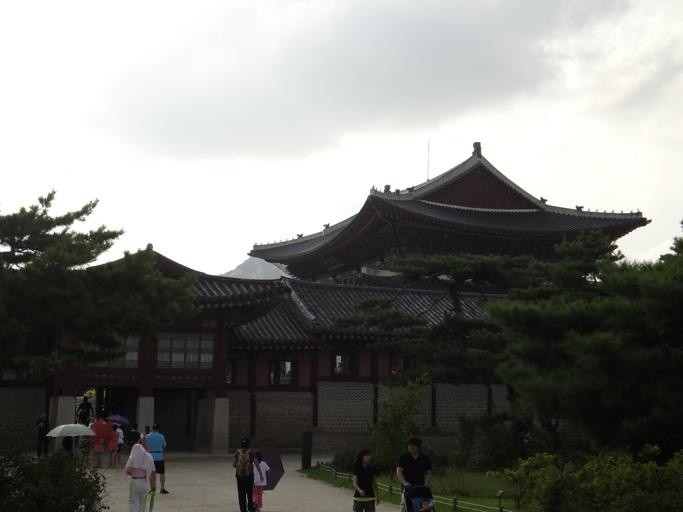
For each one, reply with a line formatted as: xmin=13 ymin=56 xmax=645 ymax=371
xmin=236 ymin=448 xmax=251 ymax=477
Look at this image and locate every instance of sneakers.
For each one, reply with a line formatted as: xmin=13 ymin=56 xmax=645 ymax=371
xmin=160 ymin=488 xmax=170 ymax=494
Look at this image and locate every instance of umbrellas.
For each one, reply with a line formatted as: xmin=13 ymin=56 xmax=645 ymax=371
xmin=46 ymin=423 xmax=97 ymax=437
xmin=254 ymin=434 xmax=284 ymax=489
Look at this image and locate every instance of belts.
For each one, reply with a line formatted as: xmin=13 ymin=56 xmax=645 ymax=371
xmin=132 ymin=476 xmax=146 ymax=480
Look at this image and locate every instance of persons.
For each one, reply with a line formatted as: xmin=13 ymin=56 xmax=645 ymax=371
xmin=418 ymin=499 xmax=433 ymax=512
xmin=351 ymin=449 xmax=379 ymax=512
xmin=396 ymin=437 xmax=432 ymax=512
xmin=74 ymin=413 xmax=151 ymax=469
xmin=252 ymin=451 xmax=270 ymax=512
xmin=76 ymin=396 xmax=95 ymax=426
xmin=232 ymin=437 xmax=264 ymax=512
xmin=144 ymin=423 xmax=169 ymax=494
xmin=35 ymin=411 xmax=51 ymax=458
xmin=54 ymin=437 xmax=74 ymax=457
xmin=124 ymin=443 xmax=156 ymax=512
